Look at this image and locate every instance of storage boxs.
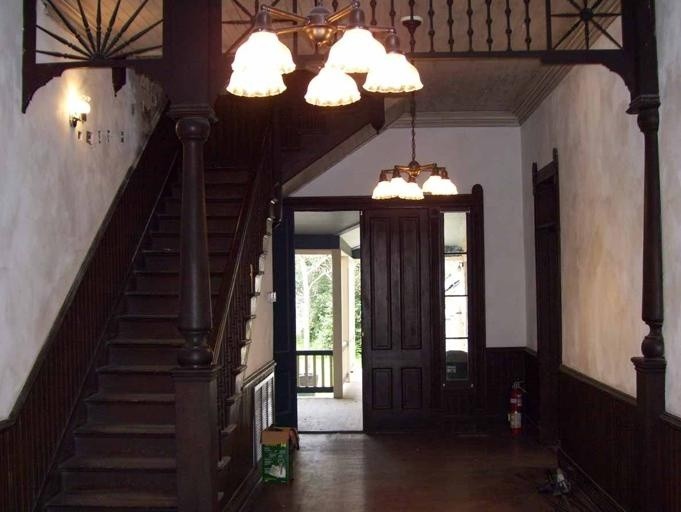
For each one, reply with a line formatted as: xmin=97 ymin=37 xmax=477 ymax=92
xmin=258 ymin=421 xmax=300 ymax=485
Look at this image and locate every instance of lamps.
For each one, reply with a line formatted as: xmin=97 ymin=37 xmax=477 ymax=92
xmin=370 ymin=15 xmax=459 ymax=204
xmin=70 ymin=92 xmax=92 ymax=129
xmin=226 ymin=0 xmax=428 ymax=109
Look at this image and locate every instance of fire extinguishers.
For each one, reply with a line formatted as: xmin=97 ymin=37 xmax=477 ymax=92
xmin=507 ymin=378 xmax=526 ymax=436
